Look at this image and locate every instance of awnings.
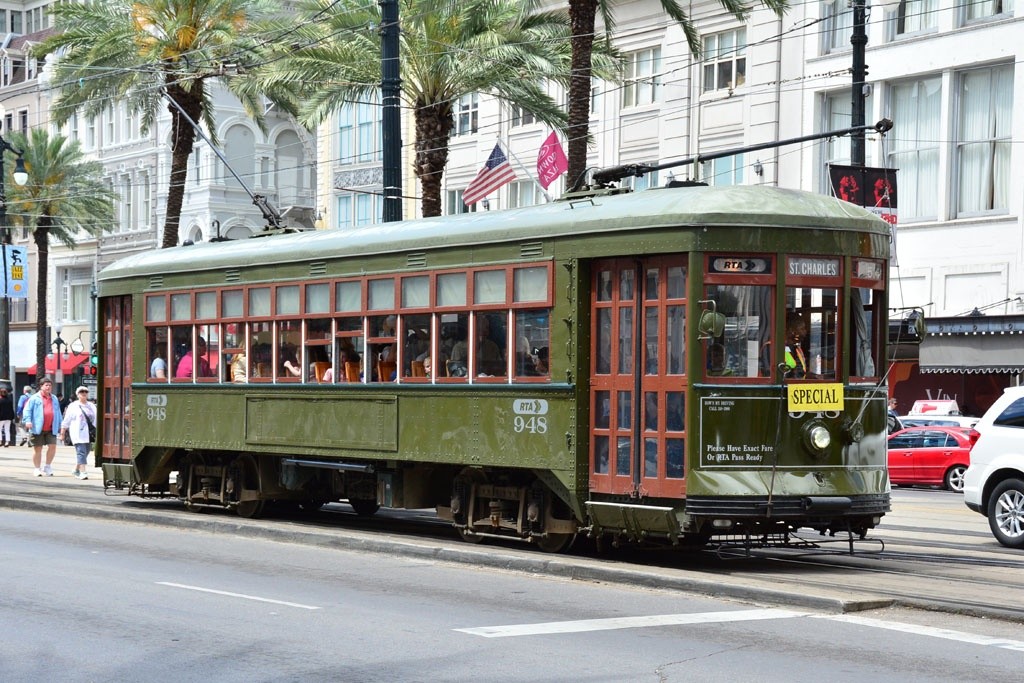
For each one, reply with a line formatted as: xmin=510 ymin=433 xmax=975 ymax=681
xmin=27 ymin=352 xmax=90 ymax=374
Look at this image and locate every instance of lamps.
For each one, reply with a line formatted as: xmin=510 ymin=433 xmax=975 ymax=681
xmin=753 ymin=158 xmax=762 ymax=177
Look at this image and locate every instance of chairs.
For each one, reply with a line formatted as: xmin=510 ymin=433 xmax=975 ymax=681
xmin=257 ymin=356 xmax=452 ymax=382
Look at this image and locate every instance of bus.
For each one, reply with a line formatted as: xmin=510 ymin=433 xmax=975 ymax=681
xmin=71 ymin=85 xmax=926 ymax=565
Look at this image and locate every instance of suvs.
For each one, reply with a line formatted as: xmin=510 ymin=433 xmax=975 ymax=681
xmin=963 ymin=384 xmax=1023 ymax=545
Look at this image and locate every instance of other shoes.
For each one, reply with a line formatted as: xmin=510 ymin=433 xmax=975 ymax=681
xmin=72 ymin=469 xmax=79 ymax=478
xmin=0 ymin=444 xmax=3 ymax=447
xmin=4 ymin=444 xmax=8 ymax=447
xmin=33 ymin=468 xmax=42 ymax=477
xmin=80 ymin=472 xmax=88 ymax=480
xmin=20 ymin=438 xmax=27 ymax=446
xmin=43 ymin=466 xmax=53 ymax=477
xmin=28 ymin=441 xmax=32 ymax=447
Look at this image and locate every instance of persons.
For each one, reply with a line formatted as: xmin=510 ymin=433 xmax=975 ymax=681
xmin=706 ymin=343 xmax=735 ymax=377
xmin=888 ymin=398 xmax=899 ymax=416
xmin=761 ymin=311 xmax=821 ymax=379
xmin=17 ymin=386 xmax=33 ymax=447
xmin=57 ymin=393 xmax=76 ymax=415
xmin=150 ymin=342 xmax=175 ymax=379
xmin=22 ymin=377 xmax=63 ymax=477
xmin=0 ymin=388 xmax=15 ymax=447
xmin=176 ymin=336 xmax=213 ymax=378
xmin=60 ymin=386 xmax=97 ymax=480
xmin=256 ymin=311 xmax=549 ymax=382
xmin=231 ymin=337 xmax=257 ymax=383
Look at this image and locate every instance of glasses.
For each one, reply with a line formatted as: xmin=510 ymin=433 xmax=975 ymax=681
xmin=79 ymin=390 xmax=87 ymax=394
xmin=894 ymin=403 xmax=898 ymax=405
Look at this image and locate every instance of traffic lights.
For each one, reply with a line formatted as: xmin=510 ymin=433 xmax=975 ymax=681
xmin=90 ymin=342 xmax=98 ymax=377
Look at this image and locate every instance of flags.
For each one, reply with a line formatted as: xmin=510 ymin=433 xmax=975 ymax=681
xmin=460 ymin=143 xmax=517 ymax=206
xmin=536 ymin=129 xmax=569 ymax=191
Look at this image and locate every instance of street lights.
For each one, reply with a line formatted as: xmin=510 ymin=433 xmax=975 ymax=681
xmin=43 ymin=324 xmax=71 ymax=403
xmin=0 ymin=102 xmax=30 ymax=447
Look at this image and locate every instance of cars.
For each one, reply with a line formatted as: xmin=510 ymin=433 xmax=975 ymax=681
xmin=893 ymin=399 xmax=983 ymax=439
xmin=882 ymin=426 xmax=979 ymax=494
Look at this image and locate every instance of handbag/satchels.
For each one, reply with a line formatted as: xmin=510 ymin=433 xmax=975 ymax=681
xmin=79 ymin=405 xmax=96 ymax=443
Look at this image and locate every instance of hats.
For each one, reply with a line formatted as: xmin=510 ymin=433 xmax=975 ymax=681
xmin=76 ymin=385 xmax=89 ymax=396
